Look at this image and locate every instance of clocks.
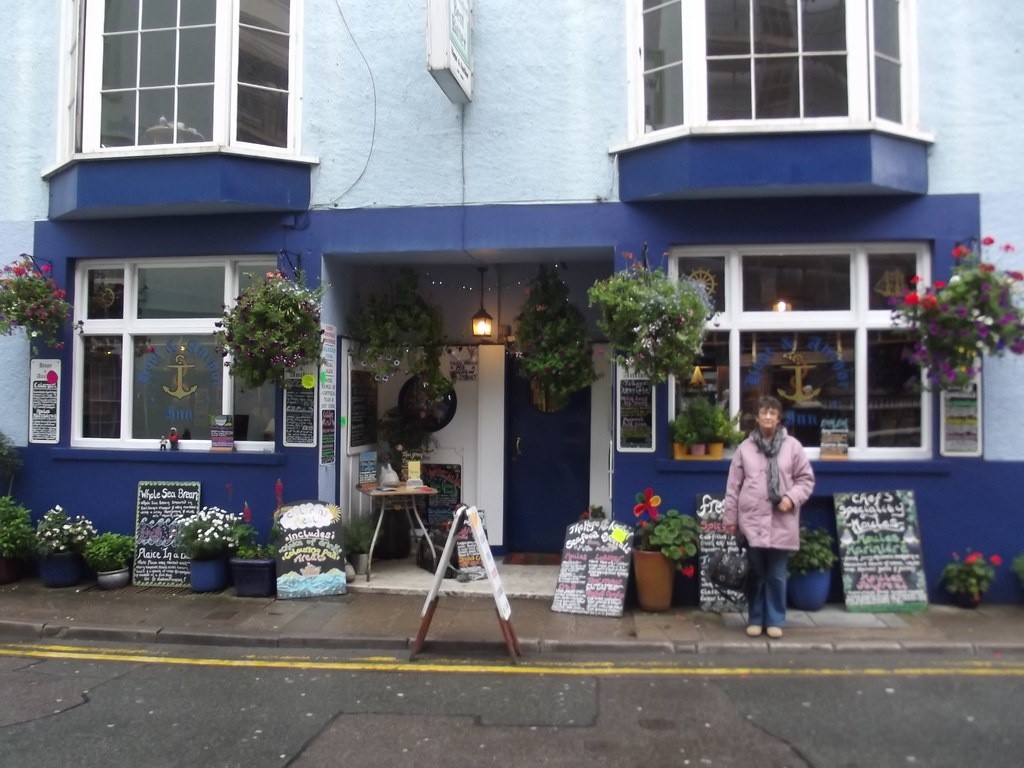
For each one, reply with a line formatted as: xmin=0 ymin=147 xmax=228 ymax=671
xmin=92 ymin=287 xmax=115 ymax=309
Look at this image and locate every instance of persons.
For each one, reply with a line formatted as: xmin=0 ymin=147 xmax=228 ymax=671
xmin=721 ymin=396 xmax=816 ymax=637
xmin=159 ymin=436 xmax=168 ymax=450
xmin=167 ymin=427 xmax=180 ymax=450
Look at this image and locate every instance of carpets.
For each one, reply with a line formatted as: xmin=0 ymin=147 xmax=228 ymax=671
xmin=503 ymin=552 xmax=562 ymax=567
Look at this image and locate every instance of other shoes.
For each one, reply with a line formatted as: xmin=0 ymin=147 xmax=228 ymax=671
xmin=746 ymin=624 xmax=763 ymax=636
xmin=767 ymin=625 xmax=783 ymax=637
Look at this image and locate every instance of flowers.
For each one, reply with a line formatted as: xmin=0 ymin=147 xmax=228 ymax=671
xmin=633 ymin=485 xmax=699 ymax=577
xmin=0 ymin=495 xmax=99 ymax=558
xmin=169 ymin=505 xmax=244 ymax=560
xmin=211 ymin=250 xmax=718 ymax=404
xmin=940 ymin=548 xmax=1002 ymax=601
xmin=580 ymin=506 xmax=605 ymax=520
xmin=887 ymin=234 xmax=1024 ymax=394
xmin=0 ymin=253 xmax=74 ymax=352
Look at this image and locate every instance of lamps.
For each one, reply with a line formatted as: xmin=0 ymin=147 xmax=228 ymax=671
xmin=472 ymin=268 xmax=494 ymax=338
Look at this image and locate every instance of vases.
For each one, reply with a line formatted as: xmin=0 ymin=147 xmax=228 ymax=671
xmin=188 ymin=558 xmax=231 ymax=592
xmin=949 ymin=592 xmax=983 ymax=609
xmin=632 ymin=545 xmax=676 ymax=612
xmin=0 ymin=552 xmax=80 ymax=588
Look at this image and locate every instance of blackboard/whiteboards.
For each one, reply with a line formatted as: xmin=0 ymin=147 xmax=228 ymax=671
xmin=274 ymin=501 xmax=347 ymax=598
xmin=283 ymin=374 xmax=315 ymax=444
xmin=211 ymin=414 xmax=235 ymax=446
xmin=134 ymin=481 xmax=203 ymax=588
xmin=348 ymin=356 xmax=379 ymax=456
xmin=831 ymin=490 xmax=930 ymax=610
xmin=550 ymin=518 xmax=633 ymax=616
xmin=30 ymin=380 xmax=58 ymax=442
xmin=456 ymin=510 xmax=490 ymax=581
xmin=941 ymin=357 xmax=983 ymax=457
xmin=467 ymin=504 xmax=514 ymax=619
xmin=617 ymin=378 xmax=653 ymax=450
xmin=420 ymin=463 xmax=462 ymax=525
xmin=695 ymin=493 xmax=749 ymax=611
xmin=323 ymin=410 xmax=337 ymax=465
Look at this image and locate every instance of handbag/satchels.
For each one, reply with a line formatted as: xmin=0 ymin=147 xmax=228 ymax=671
xmin=704 ymin=534 xmax=751 ymax=591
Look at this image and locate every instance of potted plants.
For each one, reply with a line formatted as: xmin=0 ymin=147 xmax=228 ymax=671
xmin=228 ymin=524 xmax=276 ymax=598
xmin=368 ymin=404 xmax=440 ymax=560
xmin=83 ymin=531 xmax=136 ymax=590
xmin=787 ymin=523 xmax=839 ymax=612
xmin=668 ymin=393 xmax=746 ymax=458
xmin=341 ymin=510 xmax=384 ymax=575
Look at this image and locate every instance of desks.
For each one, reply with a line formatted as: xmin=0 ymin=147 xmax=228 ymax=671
xmin=356 ymin=482 xmax=438 ymax=582
xmin=144 ymin=125 xmax=205 ymax=146
